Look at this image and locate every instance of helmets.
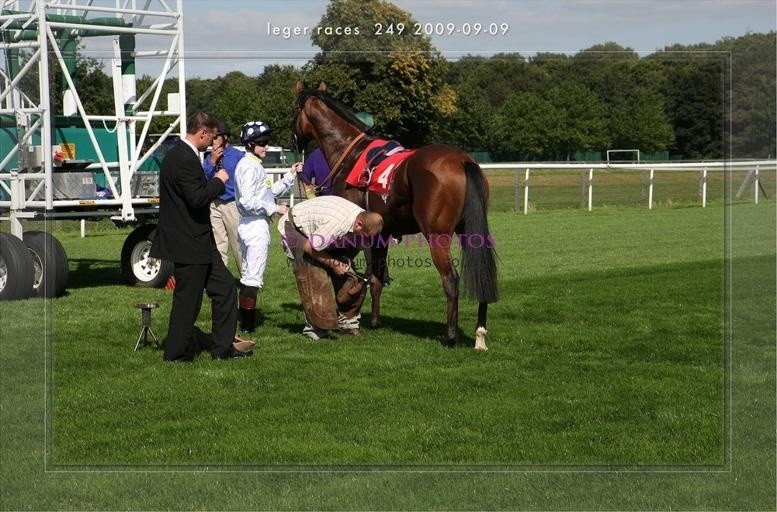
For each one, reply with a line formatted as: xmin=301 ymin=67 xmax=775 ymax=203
xmin=240 ymin=120 xmax=272 ymax=143
xmin=214 ymin=119 xmax=232 ymax=138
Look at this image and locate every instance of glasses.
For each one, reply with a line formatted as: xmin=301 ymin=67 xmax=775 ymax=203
xmin=253 ymin=140 xmax=268 ymax=147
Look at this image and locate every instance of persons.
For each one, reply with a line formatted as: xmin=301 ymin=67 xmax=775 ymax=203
xmin=198 ymin=117 xmax=245 ymax=283
xmin=232 ymin=120 xmax=304 ymax=336
xmin=275 ymin=193 xmax=384 ymax=339
xmin=143 ymin=110 xmax=255 ymax=363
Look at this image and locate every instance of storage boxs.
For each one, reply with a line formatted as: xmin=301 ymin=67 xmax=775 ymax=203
xmin=29 ymin=170 xmax=97 ymax=201
xmin=107 ymin=170 xmax=161 ymax=198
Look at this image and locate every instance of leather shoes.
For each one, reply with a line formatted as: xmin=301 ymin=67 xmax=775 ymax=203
xmin=211 ymin=345 xmax=253 ymax=360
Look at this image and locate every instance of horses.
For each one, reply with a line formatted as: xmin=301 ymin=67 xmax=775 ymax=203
xmin=288 ymin=77 xmax=501 ymax=352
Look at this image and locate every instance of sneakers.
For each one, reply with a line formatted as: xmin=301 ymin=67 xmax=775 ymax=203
xmin=301 ymin=326 xmax=364 ymax=341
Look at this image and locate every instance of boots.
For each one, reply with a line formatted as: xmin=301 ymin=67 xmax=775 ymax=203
xmin=236 ymin=307 xmax=257 ymax=334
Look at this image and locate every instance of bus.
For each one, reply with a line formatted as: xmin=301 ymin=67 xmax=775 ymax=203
xmin=200 ymin=145 xmax=292 ymax=168
